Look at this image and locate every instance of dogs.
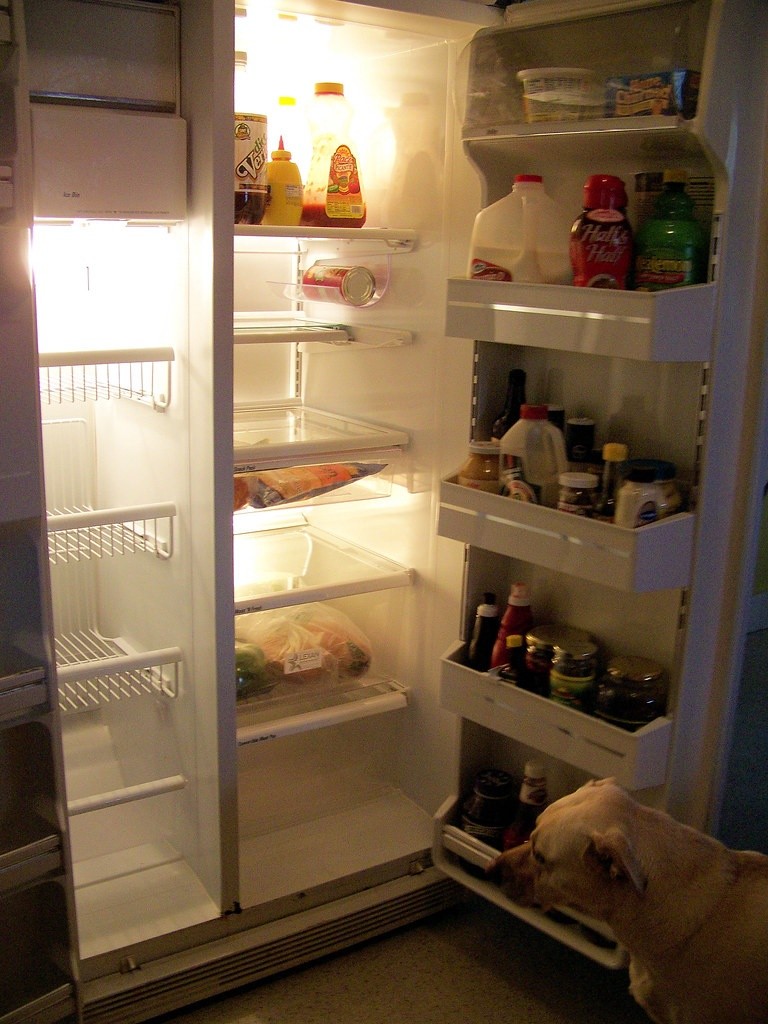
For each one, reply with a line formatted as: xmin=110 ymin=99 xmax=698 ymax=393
xmin=485 ymin=774 xmax=768 ymax=1024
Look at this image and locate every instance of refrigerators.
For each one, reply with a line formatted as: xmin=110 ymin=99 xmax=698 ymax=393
xmin=0 ymin=0 xmax=768 ymax=1024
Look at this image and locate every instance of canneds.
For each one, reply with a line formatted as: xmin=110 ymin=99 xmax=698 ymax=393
xmin=521 ymin=625 xmax=666 ymax=733
xmin=301 ymin=265 xmax=376 ymax=307
xmin=456 ymin=441 xmax=683 ymax=528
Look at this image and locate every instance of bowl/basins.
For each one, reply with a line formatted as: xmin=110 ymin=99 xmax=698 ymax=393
xmin=515 ymin=64 xmax=603 ymax=124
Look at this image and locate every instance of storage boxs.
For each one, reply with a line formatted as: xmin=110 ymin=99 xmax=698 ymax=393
xmin=605 ymin=69 xmax=701 ymax=120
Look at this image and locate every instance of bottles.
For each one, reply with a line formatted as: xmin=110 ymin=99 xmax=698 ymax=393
xmin=262 ymin=136 xmax=304 ymax=226
xmin=570 ymin=174 xmax=632 ymax=291
xmin=497 ymin=404 xmax=570 ymax=508
xmin=234 ymin=50 xmax=269 ymax=225
xmin=457 ymin=370 xmax=683 ymax=531
xmin=467 ymin=583 xmax=667 ymax=732
xmin=632 ymin=171 xmax=698 ymax=291
xmin=457 ymin=758 xmax=549 ymax=884
xmin=301 ymin=83 xmax=368 ymax=227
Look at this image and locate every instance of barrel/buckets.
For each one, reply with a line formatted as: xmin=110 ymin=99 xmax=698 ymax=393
xmin=467 ymin=172 xmax=574 ymax=285
